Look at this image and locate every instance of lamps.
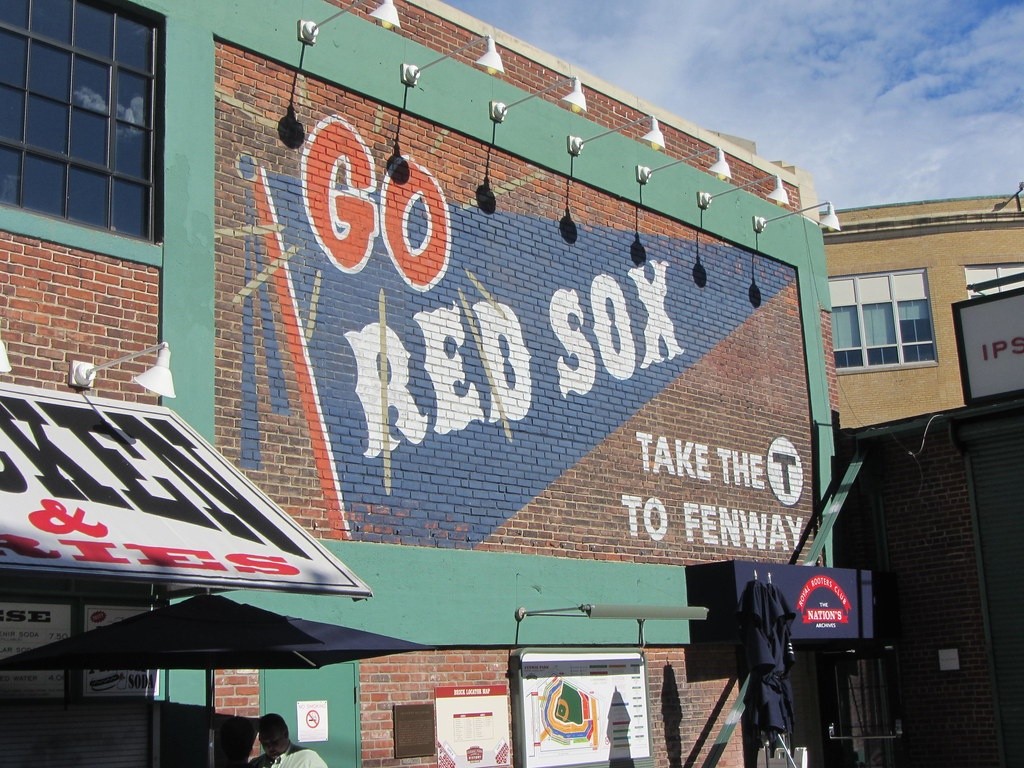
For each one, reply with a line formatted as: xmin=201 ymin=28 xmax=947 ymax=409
xmin=297 ymin=0 xmax=402 ymax=46
xmin=636 ymin=146 xmax=731 ymax=185
xmin=70 ymin=342 xmax=176 ymax=398
xmin=490 ymin=76 xmax=587 ymax=123
xmin=401 ymin=34 xmax=504 ymax=87
xmin=567 ymin=115 xmax=665 ymax=156
xmin=697 ymin=174 xmax=790 ymax=210
xmin=753 ymin=201 xmax=841 ymax=233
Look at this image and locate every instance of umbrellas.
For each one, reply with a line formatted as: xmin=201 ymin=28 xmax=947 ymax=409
xmin=0 ymin=595 xmax=436 ymax=768
xmin=734 ymin=569 xmax=796 ymax=768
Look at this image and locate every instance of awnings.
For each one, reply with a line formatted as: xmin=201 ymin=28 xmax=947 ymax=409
xmin=1 ymin=382 xmax=374 ymax=598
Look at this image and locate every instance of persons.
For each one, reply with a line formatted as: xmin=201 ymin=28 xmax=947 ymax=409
xmin=220 ymin=713 xmax=329 ymax=768
